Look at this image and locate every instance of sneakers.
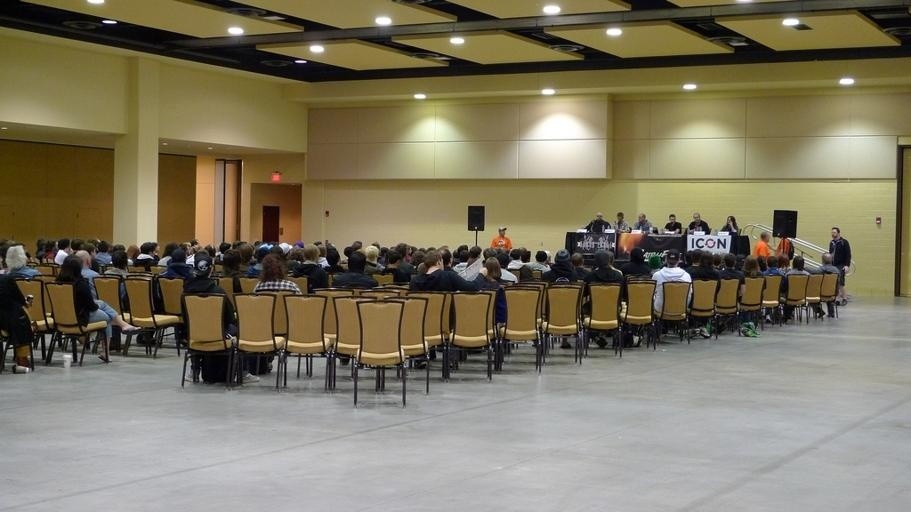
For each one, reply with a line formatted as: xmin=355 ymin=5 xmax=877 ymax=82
xmin=766 ymin=316 xmax=792 ymax=324
xmin=836 ymin=298 xmax=847 ymax=306
xmin=242 ymin=372 xmax=260 ymax=383
xmin=689 ymin=328 xmax=710 ymax=340
xmin=122 ymin=326 xmax=141 ymax=335
xmin=99 ymin=352 xmax=112 ymax=363
xmin=742 ymin=321 xmax=757 ymax=333
xmin=185 ymin=364 xmax=199 ymax=382
xmin=592 ymin=333 xmax=642 ymax=348
xmin=818 ymin=311 xmax=835 ymax=319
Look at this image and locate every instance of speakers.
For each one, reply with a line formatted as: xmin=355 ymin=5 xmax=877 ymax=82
xmin=468 ymin=205 xmax=484 ymax=231
xmin=773 ymin=210 xmax=797 ymax=238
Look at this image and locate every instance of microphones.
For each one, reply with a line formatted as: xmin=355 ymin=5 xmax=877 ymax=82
xmin=614 ymin=221 xmax=616 ymax=228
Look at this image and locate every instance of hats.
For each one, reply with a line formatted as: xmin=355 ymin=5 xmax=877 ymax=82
xmin=364 ymin=244 xmax=382 ymax=264
xmin=497 ymin=226 xmax=509 ymax=233
xmin=667 ymin=249 xmax=680 ymax=266
xmin=192 ymin=255 xmax=212 ymax=277
xmin=278 ymin=241 xmax=294 ymax=255
xmin=554 ymin=248 xmax=571 ymax=263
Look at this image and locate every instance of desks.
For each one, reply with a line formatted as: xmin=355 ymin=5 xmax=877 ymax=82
xmin=563 ymin=231 xmax=751 ymax=258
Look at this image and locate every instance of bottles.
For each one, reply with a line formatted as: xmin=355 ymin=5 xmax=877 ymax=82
xmin=640 ymin=226 xmax=643 ymax=232
xmin=659 ymin=227 xmax=663 ymax=234
xmin=602 ymin=224 xmax=614 ymax=232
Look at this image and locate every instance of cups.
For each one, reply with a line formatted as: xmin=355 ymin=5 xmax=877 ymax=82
xmin=13 ymin=365 xmax=30 ymax=372
xmin=62 ymin=354 xmax=72 ymax=369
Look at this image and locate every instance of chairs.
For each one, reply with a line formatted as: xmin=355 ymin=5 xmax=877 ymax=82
xmin=1 ymin=257 xmax=186 ymax=372
xmin=543 ymin=273 xmax=839 ymax=366
xmin=181 ymin=262 xmax=544 ymax=406
